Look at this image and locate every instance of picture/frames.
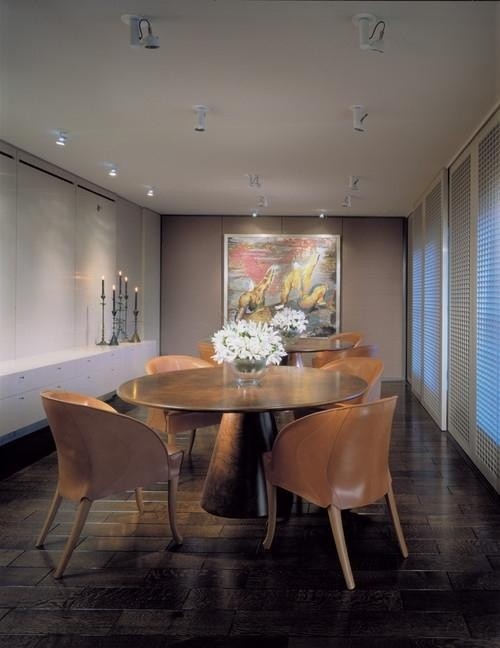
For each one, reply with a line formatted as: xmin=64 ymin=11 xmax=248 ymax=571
xmin=221 ymin=233 xmax=341 ymax=340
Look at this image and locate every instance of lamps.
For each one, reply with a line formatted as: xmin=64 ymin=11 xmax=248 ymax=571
xmin=349 ymin=104 xmax=368 ymax=132
xmin=193 ymin=105 xmax=209 ymax=131
xmin=351 ymin=13 xmax=385 ymax=53
xmin=350 ymin=175 xmax=362 ymax=192
xmin=257 ymin=195 xmax=269 ymax=207
xmin=54 ymin=128 xmax=69 ymax=146
xmin=121 ymin=13 xmax=161 ymax=52
xmin=342 ymin=193 xmax=355 ymax=208
xmin=248 ymin=172 xmax=261 ymax=188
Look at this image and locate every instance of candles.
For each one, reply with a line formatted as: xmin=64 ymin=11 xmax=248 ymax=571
xmin=100 ymin=270 xmax=139 ymax=316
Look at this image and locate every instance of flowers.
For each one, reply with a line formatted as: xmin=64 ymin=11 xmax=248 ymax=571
xmin=209 ymin=319 xmax=287 ymax=372
xmin=270 ymin=307 xmax=308 ymax=337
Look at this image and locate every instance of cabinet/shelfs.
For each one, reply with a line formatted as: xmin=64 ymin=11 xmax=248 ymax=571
xmin=0 ymin=139 xmax=160 ymax=445
